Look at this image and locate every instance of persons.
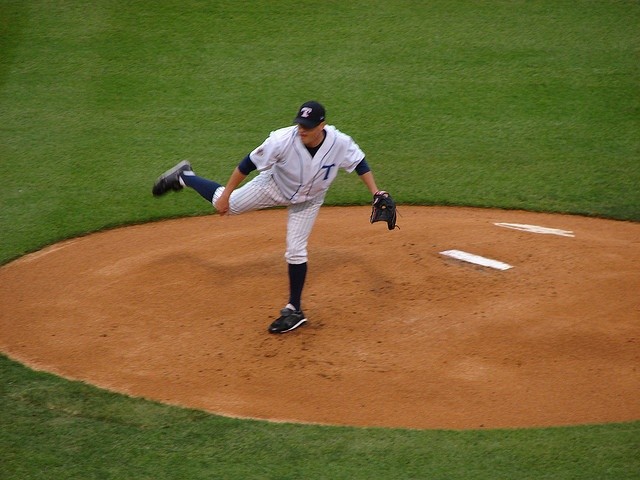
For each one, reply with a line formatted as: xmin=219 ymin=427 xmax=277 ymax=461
xmin=153 ymin=101 xmax=396 ymax=334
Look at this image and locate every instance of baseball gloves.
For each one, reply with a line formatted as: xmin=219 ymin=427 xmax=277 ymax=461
xmin=370 ymin=191 xmax=401 ymax=230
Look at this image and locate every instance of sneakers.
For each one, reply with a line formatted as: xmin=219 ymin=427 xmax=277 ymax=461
xmin=151 ymin=159 xmax=193 ymax=198
xmin=268 ymin=307 xmax=308 ymax=334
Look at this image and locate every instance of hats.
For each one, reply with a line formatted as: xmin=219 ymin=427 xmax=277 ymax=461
xmin=292 ymin=101 xmax=326 ymax=128
